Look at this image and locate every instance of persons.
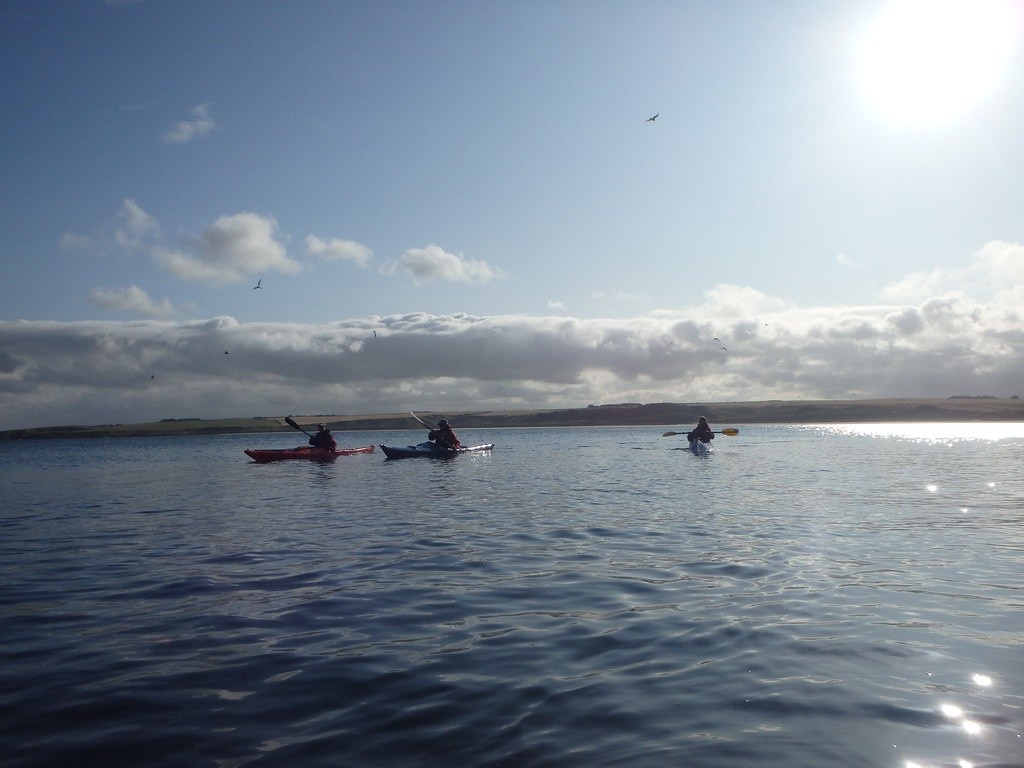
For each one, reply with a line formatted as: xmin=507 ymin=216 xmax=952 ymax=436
xmin=687 ymin=416 xmax=715 ymax=443
xmin=308 ymin=422 xmax=337 ymax=452
xmin=428 ymin=419 xmax=460 ymax=449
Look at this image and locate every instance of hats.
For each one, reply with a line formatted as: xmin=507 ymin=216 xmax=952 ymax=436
xmin=438 ymin=419 xmax=448 ymax=426
xmin=317 ymin=422 xmax=326 ymax=427
xmin=699 ymin=416 xmax=706 ymax=421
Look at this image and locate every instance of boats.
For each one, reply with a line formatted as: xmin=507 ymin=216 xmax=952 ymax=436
xmin=379 ymin=441 xmax=460 ymax=460
xmin=244 ymin=444 xmax=375 ymax=462
xmin=689 ymin=436 xmax=712 ymax=456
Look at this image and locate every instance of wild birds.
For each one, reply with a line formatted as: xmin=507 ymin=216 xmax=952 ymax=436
xmin=251 ymin=280 xmax=263 ymax=292
xmin=643 ymin=112 xmax=659 ymax=122
xmin=711 ymin=322 xmax=771 ymax=352
xmin=372 ymin=330 xmax=378 ymax=340
xmin=223 ymin=349 xmax=230 ymax=355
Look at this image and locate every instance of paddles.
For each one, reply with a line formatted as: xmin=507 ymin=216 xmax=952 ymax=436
xmin=410 ymin=411 xmax=460 ymax=454
xmin=285 ymin=416 xmax=334 ymax=453
xmin=663 ymin=427 xmax=739 ymax=437
